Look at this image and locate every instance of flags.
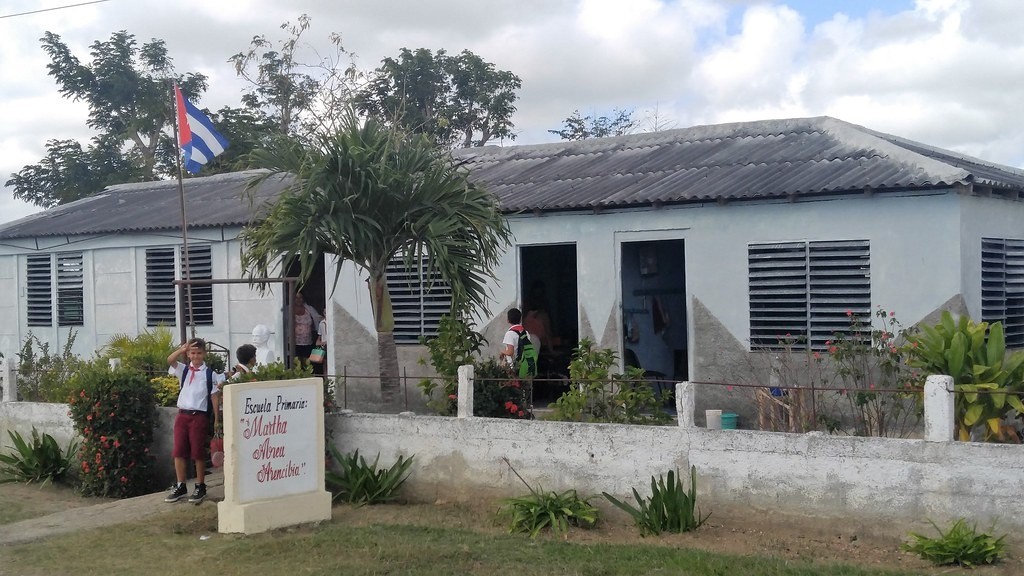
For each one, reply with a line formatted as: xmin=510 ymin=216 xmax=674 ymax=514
xmin=174 ymin=81 xmax=230 ymax=174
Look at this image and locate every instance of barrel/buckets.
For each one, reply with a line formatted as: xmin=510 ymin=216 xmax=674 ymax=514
xmin=705 ymin=410 xmax=722 ymax=429
xmin=721 ymin=414 xmax=739 ymax=429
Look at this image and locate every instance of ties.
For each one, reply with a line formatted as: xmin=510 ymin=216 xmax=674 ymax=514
xmin=188 ymin=365 xmax=200 ymax=386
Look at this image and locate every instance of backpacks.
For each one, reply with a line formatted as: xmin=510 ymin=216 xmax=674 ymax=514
xmin=509 ymin=328 xmax=538 ymax=379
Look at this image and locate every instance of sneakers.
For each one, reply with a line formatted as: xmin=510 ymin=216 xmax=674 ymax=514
xmin=189 ymin=482 xmax=207 ymax=501
xmin=164 ymin=482 xmax=188 ymax=502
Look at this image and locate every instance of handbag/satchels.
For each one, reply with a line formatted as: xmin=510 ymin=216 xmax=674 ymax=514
xmin=210 ymin=433 xmax=224 ymax=468
xmin=309 ymin=346 xmax=326 ymax=364
xmin=497 ymin=354 xmax=506 ymax=367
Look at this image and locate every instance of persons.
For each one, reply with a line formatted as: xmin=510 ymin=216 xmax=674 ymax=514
xmin=216 ymin=344 xmax=257 ymax=391
xmin=286 ymin=293 xmax=323 ymax=369
xmin=165 ymin=337 xmax=219 ymax=502
xmin=316 ymin=308 xmax=326 ymax=374
xmin=499 ymin=309 xmax=530 ymax=407
